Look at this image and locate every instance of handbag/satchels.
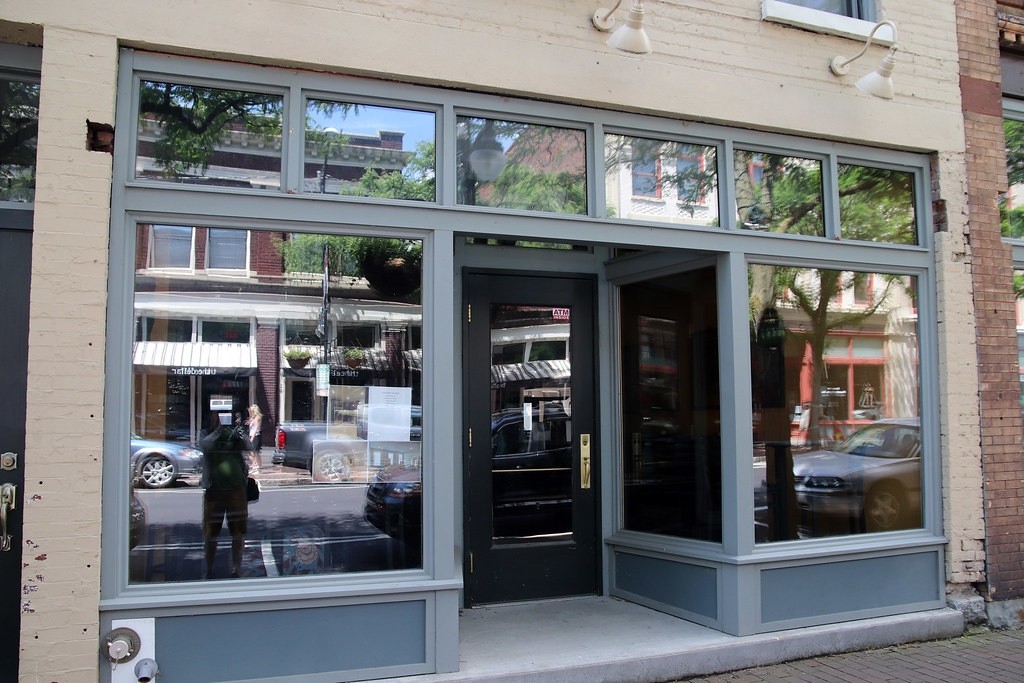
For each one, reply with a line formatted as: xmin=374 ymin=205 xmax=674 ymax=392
xmin=247 ymin=477 xmax=260 ymax=504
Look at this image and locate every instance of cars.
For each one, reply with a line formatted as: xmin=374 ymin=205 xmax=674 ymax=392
xmin=129 ymin=431 xmax=206 ymax=491
xmin=273 ymin=422 xmax=366 ymax=482
xmin=763 ymin=415 xmax=927 ymax=537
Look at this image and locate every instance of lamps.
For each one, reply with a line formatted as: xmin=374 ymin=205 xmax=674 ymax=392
xmin=593 ymin=0 xmax=653 ymax=56
xmin=831 ymin=21 xmax=900 ymax=100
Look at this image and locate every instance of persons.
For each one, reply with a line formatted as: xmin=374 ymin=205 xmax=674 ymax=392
xmin=895 ymin=434 xmax=920 ymax=460
xmin=202 ymin=405 xmax=262 ymax=580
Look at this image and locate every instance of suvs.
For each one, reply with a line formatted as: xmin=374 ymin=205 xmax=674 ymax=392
xmin=363 ymin=402 xmax=580 ymax=546
